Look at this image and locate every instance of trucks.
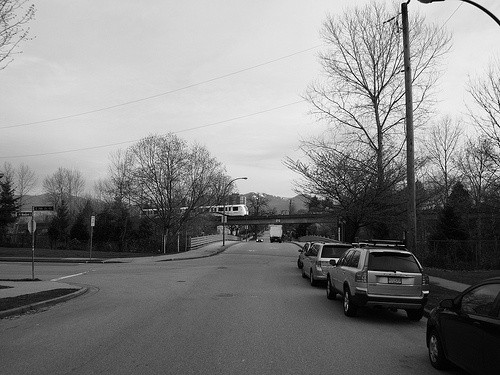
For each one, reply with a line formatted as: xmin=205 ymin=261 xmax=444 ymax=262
xmin=269 ymin=225 xmax=283 ymax=243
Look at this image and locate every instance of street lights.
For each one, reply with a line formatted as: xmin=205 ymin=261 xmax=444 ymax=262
xmin=222 ymin=178 xmax=247 ymax=246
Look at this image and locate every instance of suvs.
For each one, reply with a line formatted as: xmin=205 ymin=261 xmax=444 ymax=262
xmin=302 ymin=241 xmax=352 ymax=286
xmin=326 ymin=238 xmax=429 ymax=321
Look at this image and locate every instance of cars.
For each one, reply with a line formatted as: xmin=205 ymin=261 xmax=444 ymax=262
xmin=256 ymin=238 xmax=263 ymax=242
xmin=426 ymin=277 xmax=500 ymax=375
xmin=297 ymin=242 xmax=315 ymax=269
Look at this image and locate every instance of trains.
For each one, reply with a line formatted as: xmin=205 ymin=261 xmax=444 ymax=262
xmin=140 ymin=204 xmax=249 ymax=219
